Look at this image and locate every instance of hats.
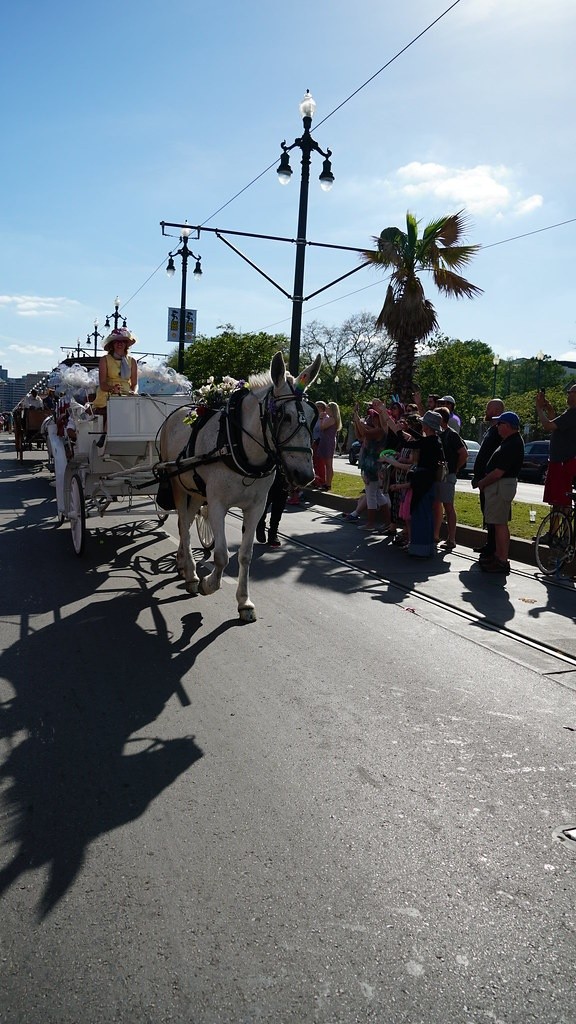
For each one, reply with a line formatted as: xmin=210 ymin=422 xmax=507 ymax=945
xmin=418 ymin=410 xmax=443 ymax=432
xmin=491 ymin=410 xmax=520 ymax=428
xmin=437 ymin=395 xmax=455 ymax=404
xmin=29 ymin=388 xmax=39 ymax=394
xmin=104 ymin=331 xmax=135 ymax=351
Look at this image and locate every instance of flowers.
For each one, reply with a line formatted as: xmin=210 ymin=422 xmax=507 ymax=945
xmin=182 ymin=375 xmax=249 ymax=426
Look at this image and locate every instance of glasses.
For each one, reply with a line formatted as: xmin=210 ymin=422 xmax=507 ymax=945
xmin=567 ymin=390 xmax=576 ymax=394
xmin=405 ymin=408 xmax=414 ymax=412
xmin=496 ymin=421 xmax=506 ymax=426
xmin=391 ymin=406 xmax=400 ymax=410
xmin=440 ymin=402 xmax=451 ymax=406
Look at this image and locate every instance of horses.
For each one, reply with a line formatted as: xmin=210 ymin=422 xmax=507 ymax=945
xmin=160 ymin=348 xmax=323 ymax=623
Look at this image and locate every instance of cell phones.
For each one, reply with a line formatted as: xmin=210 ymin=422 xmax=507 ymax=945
xmin=356 ymin=404 xmax=359 ymax=411
xmin=541 ymin=387 xmax=545 ymax=394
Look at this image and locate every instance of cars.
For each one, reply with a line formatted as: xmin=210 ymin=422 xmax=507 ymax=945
xmin=517 ymin=441 xmax=552 ymax=484
xmin=349 ymin=439 xmax=363 ymax=464
xmin=456 ymin=440 xmax=482 ymax=480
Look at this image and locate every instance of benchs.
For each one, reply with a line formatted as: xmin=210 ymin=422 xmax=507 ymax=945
xmin=54 ymin=388 xmax=105 ymax=439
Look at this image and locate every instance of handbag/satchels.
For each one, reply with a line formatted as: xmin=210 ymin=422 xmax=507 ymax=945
xmin=438 ymin=461 xmax=449 ymax=482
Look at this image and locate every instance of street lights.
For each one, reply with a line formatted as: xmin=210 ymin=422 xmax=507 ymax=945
xmin=70 ymin=337 xmax=86 ymax=358
xmin=354 ymin=372 xmax=359 ymax=397
xmin=494 ymin=354 xmax=500 ymax=399
xmin=532 ymin=348 xmax=544 ymax=441
xmin=85 ymin=316 xmax=106 ymax=358
xmin=316 ymin=377 xmax=322 ymax=403
xmin=469 ymin=414 xmax=476 ymax=442
xmin=165 ymin=221 xmax=204 ymax=374
xmin=103 ymin=295 xmax=129 ymax=332
xmin=275 ymin=86 xmax=335 ymax=377
xmin=334 ymin=375 xmax=339 ymax=404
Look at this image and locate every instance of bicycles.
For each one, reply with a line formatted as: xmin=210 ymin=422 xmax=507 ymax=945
xmin=530 ymin=493 xmax=576 ymax=576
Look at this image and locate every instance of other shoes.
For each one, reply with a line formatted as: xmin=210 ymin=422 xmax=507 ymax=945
xmin=306 ymin=481 xmax=320 ymax=490
xmin=287 ymin=496 xmax=300 ymax=504
xmin=532 ymin=532 xmax=558 ymax=545
xmin=318 ymin=483 xmax=331 ymax=491
xmin=550 ymin=536 xmax=569 ymax=550
xmin=96 ymin=434 xmax=105 ymax=447
xmin=479 ymin=555 xmax=510 ymax=574
xmin=472 ymin=543 xmax=496 ymax=555
xmin=339 ymin=510 xmax=456 ymax=557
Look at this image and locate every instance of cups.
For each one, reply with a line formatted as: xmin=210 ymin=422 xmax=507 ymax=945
xmin=530 ymin=511 xmax=536 ymax=522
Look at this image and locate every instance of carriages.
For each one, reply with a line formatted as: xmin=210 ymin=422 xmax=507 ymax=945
xmin=13 ymin=381 xmax=62 ymax=464
xmin=40 ymin=350 xmax=321 ymax=623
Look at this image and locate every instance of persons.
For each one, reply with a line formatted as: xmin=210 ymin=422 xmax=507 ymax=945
xmin=532 ymin=383 xmax=576 ymax=548
xmin=351 ymin=391 xmax=469 ymax=558
xmin=472 ymin=399 xmax=518 ymax=553
xmin=256 ymin=468 xmax=287 ymax=545
xmin=0 ymin=412 xmax=15 ymax=434
xmin=43 ymin=386 xmax=61 ymax=411
xmin=24 ymin=388 xmax=45 ymax=412
xmin=91 ymin=329 xmax=138 ymax=449
xmin=314 ymin=401 xmax=343 ymax=491
xmin=478 ymin=412 xmax=525 ymax=574
